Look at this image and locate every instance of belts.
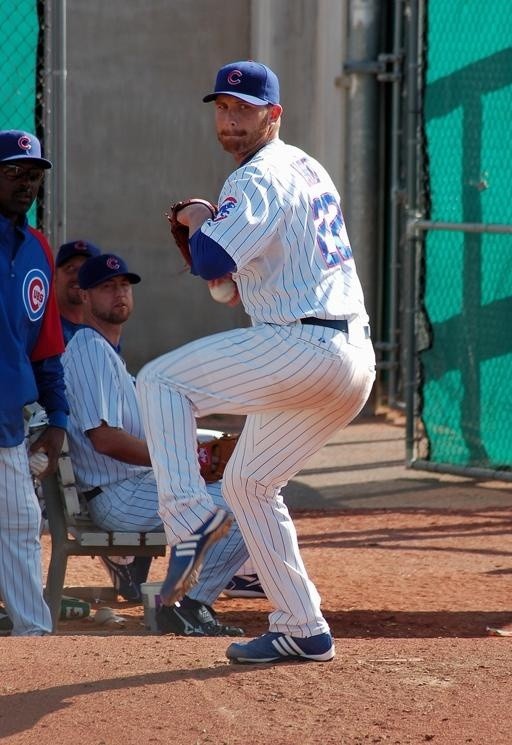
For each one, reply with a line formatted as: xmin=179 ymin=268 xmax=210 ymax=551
xmin=291 ymin=316 xmax=372 ymax=342
xmin=80 ymin=485 xmax=105 ymax=504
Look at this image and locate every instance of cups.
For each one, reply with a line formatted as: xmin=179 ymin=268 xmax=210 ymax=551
xmin=60 ymin=597 xmax=90 ymax=618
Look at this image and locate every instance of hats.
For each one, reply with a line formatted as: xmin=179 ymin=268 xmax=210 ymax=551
xmin=78 ymin=252 xmax=142 ymax=289
xmin=1 ymin=129 xmax=54 ymax=171
xmin=55 ymin=240 xmax=103 ymax=267
xmin=201 ymin=60 xmax=282 ymax=108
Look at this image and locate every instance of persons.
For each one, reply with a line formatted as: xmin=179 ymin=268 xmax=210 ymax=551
xmin=53 ymin=237 xmax=268 ymax=606
xmin=1 ymin=130 xmax=71 ymax=637
xmin=136 ymin=59 xmax=377 ymax=664
xmin=60 ymin=252 xmax=250 ymax=634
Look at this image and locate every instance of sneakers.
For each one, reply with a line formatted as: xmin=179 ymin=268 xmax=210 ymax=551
xmin=99 ymin=555 xmax=142 ymax=602
xmin=166 ymin=596 xmax=244 ymax=638
xmin=225 ymin=630 xmax=336 ymax=665
xmin=158 ymin=508 xmax=232 ymax=606
xmin=223 ymin=572 xmax=266 ymax=599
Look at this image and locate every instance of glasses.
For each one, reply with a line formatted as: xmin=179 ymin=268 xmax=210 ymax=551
xmin=1 ymin=164 xmax=45 ymax=182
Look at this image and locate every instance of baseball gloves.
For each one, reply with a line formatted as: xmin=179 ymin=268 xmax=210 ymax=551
xmin=170 ymin=199 xmax=218 ymax=267
xmin=198 ymin=433 xmax=241 ymax=483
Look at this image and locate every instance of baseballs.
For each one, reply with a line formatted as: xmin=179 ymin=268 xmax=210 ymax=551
xmin=95 ymin=607 xmax=115 ymax=625
xmin=29 ymin=453 xmax=48 ymax=476
xmin=209 ymin=279 xmax=236 ymax=303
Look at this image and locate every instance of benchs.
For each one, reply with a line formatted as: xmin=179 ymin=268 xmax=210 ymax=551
xmin=30 ymin=423 xmax=169 ymax=634
